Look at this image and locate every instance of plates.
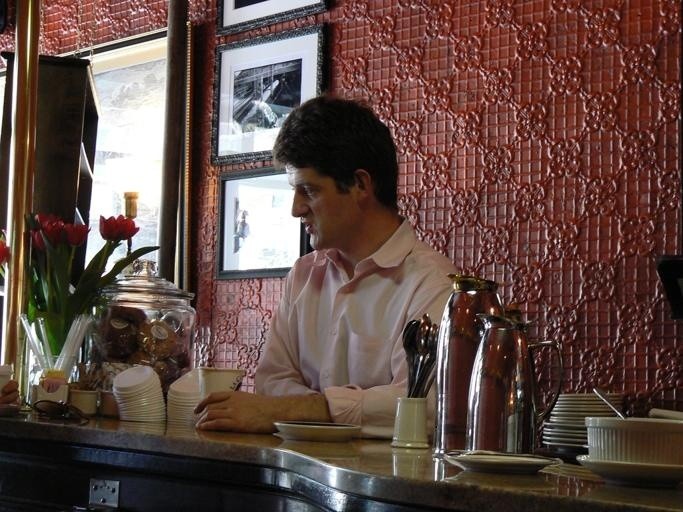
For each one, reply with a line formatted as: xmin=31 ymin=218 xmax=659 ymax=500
xmin=574 ymin=455 xmax=682 ymax=487
xmin=539 ymin=461 xmax=603 ymax=486
xmin=442 ymin=453 xmax=553 ymax=474
xmin=440 ymin=469 xmax=561 ymax=493
xmin=272 ymin=421 xmax=360 ymax=441
xmin=541 ymin=391 xmax=626 ymax=454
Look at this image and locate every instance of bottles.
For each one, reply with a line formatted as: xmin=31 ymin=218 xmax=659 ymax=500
xmin=391 ymin=451 xmax=426 ymax=481
xmin=38 ymin=368 xmax=67 ymax=392
xmin=84 ymin=259 xmax=196 ymax=402
xmin=390 ymin=397 xmax=430 ymax=450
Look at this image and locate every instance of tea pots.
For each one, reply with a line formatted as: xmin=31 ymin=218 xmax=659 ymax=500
xmin=433 ymin=272 xmax=506 ymax=458
xmin=463 ymin=311 xmax=563 ymax=456
xmin=435 ymin=458 xmax=461 ymax=482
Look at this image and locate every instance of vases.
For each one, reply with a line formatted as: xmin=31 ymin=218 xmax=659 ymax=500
xmin=28 ymin=354 xmax=77 ymax=407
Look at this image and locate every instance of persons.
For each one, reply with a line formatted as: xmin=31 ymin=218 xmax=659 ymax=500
xmin=190 ymin=95 xmax=466 ymax=435
xmin=0 ymin=377 xmax=21 ymax=406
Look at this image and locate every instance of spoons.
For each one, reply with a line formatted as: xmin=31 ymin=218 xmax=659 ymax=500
xmin=398 ymin=312 xmax=440 ymax=399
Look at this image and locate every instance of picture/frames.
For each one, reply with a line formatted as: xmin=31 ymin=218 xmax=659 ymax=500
xmin=216 ymin=0 xmax=327 ymax=39
xmin=0 ymin=21 xmax=193 ymax=294
xmin=216 ymin=167 xmax=312 ymax=280
xmin=211 ymin=23 xmax=325 ymax=166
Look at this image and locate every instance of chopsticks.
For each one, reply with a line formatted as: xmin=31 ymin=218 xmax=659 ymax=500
xmin=275 ymin=440 xmax=362 ymax=463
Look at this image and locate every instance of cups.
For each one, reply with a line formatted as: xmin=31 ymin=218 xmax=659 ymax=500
xmin=28 ymin=354 xmax=79 ymax=407
xmin=199 ymin=367 xmax=246 ymax=404
xmin=97 ymin=388 xmax=118 ymax=416
xmin=0 ymin=364 xmax=14 ymax=398
xmin=68 ymin=388 xmax=98 ymax=416
xmin=582 ymin=415 xmax=682 ymax=464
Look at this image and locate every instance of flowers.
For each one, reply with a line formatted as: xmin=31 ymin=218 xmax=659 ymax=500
xmin=0 ymin=211 xmax=160 ymax=365
